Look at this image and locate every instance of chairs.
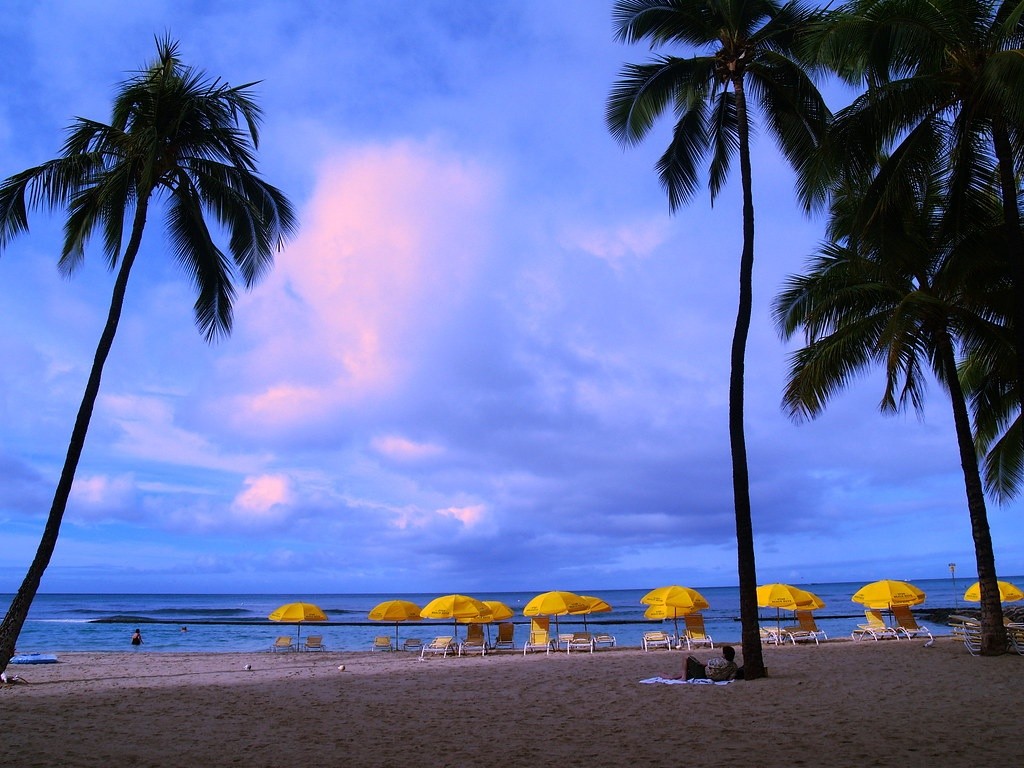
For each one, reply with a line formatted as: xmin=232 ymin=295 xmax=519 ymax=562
xmin=494 ymin=622 xmax=515 ymax=650
xmin=679 ymin=614 xmax=714 ymax=650
xmin=594 ymin=632 xmax=617 ymax=647
xmin=269 ymin=636 xmax=296 ymax=653
xmin=567 ymin=632 xmax=594 ymax=653
xmin=851 ymin=604 xmax=931 ymax=641
xmin=402 ymin=638 xmax=423 ymax=652
xmin=559 ymin=633 xmax=574 ymax=644
xmin=421 ymin=636 xmax=455 ymax=658
xmin=759 ymin=611 xmax=828 ymax=645
xmin=524 ymin=616 xmax=555 ymax=655
xmin=458 ymin=624 xmax=489 ymax=657
xmin=302 ymin=635 xmax=326 ymax=652
xmin=640 ymin=630 xmax=676 ymax=652
xmin=446 ymin=643 xmax=460 ymax=653
xmin=947 ymin=604 xmax=1024 ymax=657
xmin=371 ymin=636 xmax=393 ymax=653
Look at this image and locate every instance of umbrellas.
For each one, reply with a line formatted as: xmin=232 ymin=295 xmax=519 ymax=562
xmin=419 ymin=593 xmax=494 ymax=653
xmin=555 ymin=595 xmax=610 ymax=636
xmin=640 ymin=584 xmax=711 ymax=640
xmin=645 ymin=605 xmax=704 ymax=640
xmin=457 ymin=599 xmax=514 ymax=650
xmin=367 ymin=600 xmax=422 ymax=652
xmin=851 ymin=580 xmax=927 ymax=629
xmin=964 ymin=579 xmax=1024 ymax=606
xmin=756 ymin=582 xmax=811 ymax=642
xmin=776 ymin=590 xmax=828 ymax=627
xmin=267 ymin=603 xmax=327 ymax=653
xmin=523 ymin=591 xmax=591 ymax=650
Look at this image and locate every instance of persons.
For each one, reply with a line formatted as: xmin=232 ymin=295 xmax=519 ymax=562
xmin=180 ymin=625 xmax=188 ymax=633
xmin=659 ymin=645 xmax=740 ymax=681
xmin=131 ymin=627 xmax=145 ymax=645
